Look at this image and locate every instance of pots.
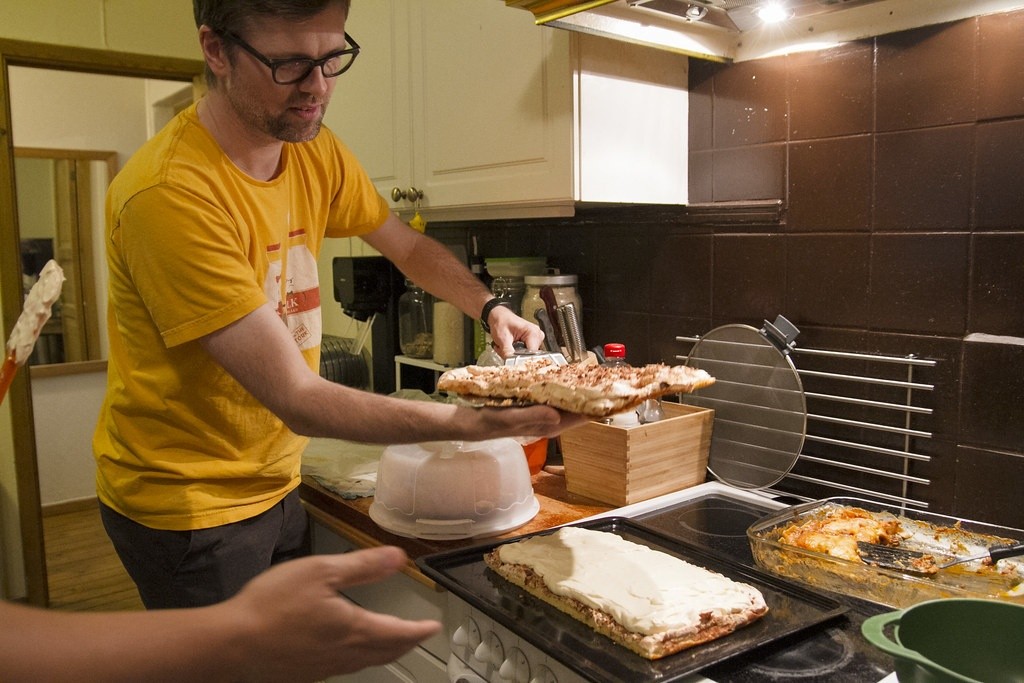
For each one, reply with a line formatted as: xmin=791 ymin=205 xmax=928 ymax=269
xmin=861 ymin=597 xmax=1024 ymax=683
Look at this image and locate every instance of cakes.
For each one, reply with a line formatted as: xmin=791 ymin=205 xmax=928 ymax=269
xmin=483 ymin=527 xmax=769 ymax=660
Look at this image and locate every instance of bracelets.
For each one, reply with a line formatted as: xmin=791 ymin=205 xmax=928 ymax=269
xmin=481 ymin=298 xmax=516 ymax=333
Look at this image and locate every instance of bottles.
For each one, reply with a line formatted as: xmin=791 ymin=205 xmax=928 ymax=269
xmin=640 ymin=395 xmax=667 ymax=425
xmin=433 ymin=293 xmax=465 ymax=364
xmin=398 ymin=278 xmax=435 ymax=358
xmin=602 ymin=343 xmax=638 ymax=368
xmin=520 ymin=274 xmax=581 ymax=348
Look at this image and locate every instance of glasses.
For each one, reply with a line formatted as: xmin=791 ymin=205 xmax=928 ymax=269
xmin=218 ymin=29 xmax=361 ymax=85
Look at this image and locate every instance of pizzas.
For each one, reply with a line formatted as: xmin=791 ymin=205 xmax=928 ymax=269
xmin=437 ymin=360 xmax=716 ymax=415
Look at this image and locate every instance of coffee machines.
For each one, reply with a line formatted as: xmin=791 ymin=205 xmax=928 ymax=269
xmin=329 ymin=253 xmax=431 ymax=393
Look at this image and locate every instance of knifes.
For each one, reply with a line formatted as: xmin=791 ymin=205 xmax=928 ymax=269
xmin=534 ymin=286 xmax=588 ymax=365
xmin=512 ymin=339 xmax=536 ymax=356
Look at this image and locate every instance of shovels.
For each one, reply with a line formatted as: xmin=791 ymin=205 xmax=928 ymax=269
xmin=0 ymin=259 xmax=66 ymax=406
xmin=856 ymin=541 xmax=1024 ymax=573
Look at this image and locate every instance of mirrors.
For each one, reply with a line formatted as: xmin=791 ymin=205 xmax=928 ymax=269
xmin=13 ymin=146 xmax=117 ymax=378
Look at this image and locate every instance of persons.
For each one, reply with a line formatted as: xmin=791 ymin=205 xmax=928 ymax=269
xmin=94 ymin=0 xmax=593 ymax=612
xmin=0 ymin=546 xmax=442 ymax=683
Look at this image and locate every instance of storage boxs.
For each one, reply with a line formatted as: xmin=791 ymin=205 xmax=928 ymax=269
xmin=556 ymin=398 xmax=716 ymax=509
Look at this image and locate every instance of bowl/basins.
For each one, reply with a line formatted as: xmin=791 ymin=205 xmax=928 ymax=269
xmin=371 ymin=440 xmax=539 ymax=540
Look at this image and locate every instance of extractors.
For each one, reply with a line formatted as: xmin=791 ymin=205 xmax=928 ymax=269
xmin=497 ymin=0 xmax=1024 ymax=70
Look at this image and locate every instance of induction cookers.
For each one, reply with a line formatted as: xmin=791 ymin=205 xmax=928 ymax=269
xmin=449 ymin=479 xmax=1024 ymax=683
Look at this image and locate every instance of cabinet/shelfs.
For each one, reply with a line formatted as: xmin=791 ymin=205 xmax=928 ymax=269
xmin=303 ymin=513 xmax=588 ymax=683
xmin=321 ymin=0 xmax=690 ymax=225
